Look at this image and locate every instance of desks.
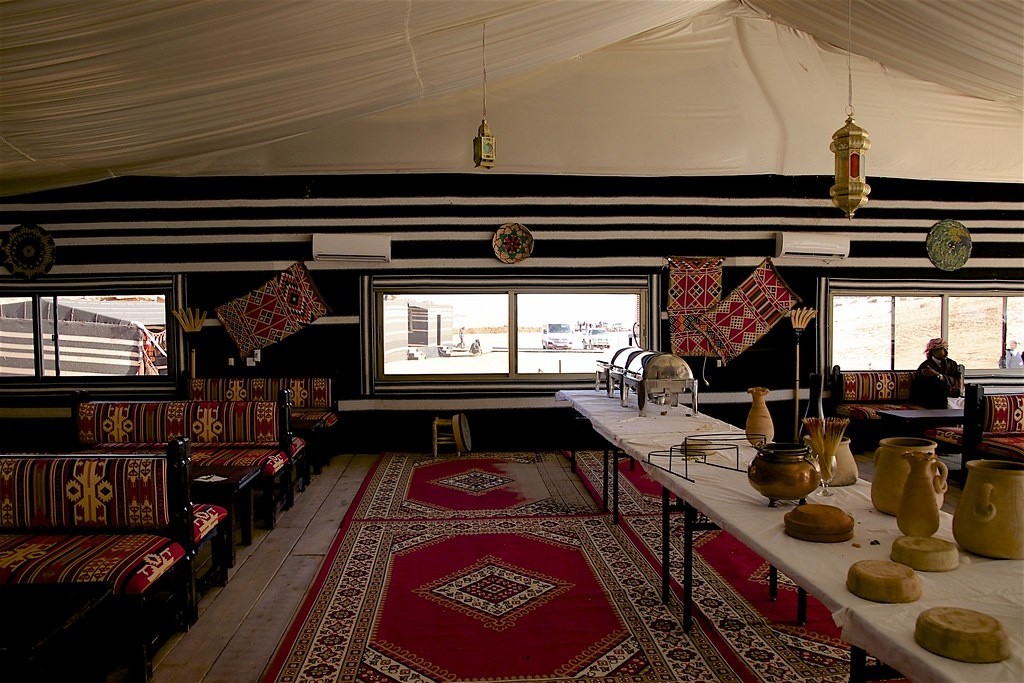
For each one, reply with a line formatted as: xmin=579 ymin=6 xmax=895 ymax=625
xmin=875 ymin=409 xmax=964 ymax=438
xmin=554 ymin=390 xmax=1024 ymax=683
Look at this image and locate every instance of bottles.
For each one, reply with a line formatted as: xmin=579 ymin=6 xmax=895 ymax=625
xmin=953 ymin=460 xmax=1024 ymax=560
xmin=804 ymin=435 xmax=859 ymax=487
xmin=870 ymin=437 xmax=949 ymax=517
xmin=798 ymin=373 xmax=825 ymax=445
xmin=897 ymin=451 xmax=949 ymax=537
xmin=748 ymin=386 xmax=774 ymax=447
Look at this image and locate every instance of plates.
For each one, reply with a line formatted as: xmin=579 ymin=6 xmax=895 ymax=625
xmin=1 ymin=222 xmax=56 ymax=281
xmin=926 ymin=219 xmax=972 ymax=271
xmin=492 ymin=223 xmax=535 ymax=264
xmin=785 ymin=504 xmax=855 ymax=542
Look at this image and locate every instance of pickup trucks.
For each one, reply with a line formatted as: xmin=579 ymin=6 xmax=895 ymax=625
xmin=581 ymin=328 xmax=612 ymax=349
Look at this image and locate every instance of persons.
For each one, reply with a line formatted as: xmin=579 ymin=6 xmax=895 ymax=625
xmin=915 ymin=338 xmax=961 ymax=410
xmin=998 ymin=341 xmax=1023 ymax=369
xmin=456 ymin=327 xmax=465 ymax=349
xmin=469 ymin=340 xmax=482 ymax=355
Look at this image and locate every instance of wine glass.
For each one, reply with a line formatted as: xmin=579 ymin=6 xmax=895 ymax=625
xmin=815 ymin=455 xmax=838 ymax=497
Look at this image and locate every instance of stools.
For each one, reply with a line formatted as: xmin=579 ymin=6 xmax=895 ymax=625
xmin=432 ymin=413 xmax=472 ymax=460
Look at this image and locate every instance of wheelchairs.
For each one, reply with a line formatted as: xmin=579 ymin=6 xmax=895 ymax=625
xmin=469 ymin=346 xmax=482 ymax=356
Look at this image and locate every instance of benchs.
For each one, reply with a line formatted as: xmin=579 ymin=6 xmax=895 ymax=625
xmin=0 ymin=377 xmax=340 ymax=683
xmin=830 ymin=365 xmax=1024 ymax=491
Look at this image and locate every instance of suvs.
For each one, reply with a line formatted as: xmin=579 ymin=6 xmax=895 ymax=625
xmin=542 ymin=323 xmax=574 ymax=350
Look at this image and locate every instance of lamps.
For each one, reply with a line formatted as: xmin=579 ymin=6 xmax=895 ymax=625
xmin=829 ymin=0 xmax=872 ymax=223
xmin=473 ymin=25 xmax=496 ymax=169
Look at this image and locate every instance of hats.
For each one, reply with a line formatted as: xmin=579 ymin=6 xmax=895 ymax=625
xmin=925 ymin=338 xmax=947 ymax=352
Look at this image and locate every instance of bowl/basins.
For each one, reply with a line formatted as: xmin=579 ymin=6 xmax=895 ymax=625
xmin=915 ymin=606 xmax=1010 ymax=662
xmin=680 ymin=440 xmax=717 ymax=455
xmin=890 ymin=535 xmax=959 ymax=572
xmin=846 ymin=560 xmax=923 ymax=603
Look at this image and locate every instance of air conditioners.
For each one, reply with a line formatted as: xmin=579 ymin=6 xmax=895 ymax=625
xmin=776 ymin=232 xmax=850 ymax=260
xmin=313 ymin=233 xmax=391 ymax=263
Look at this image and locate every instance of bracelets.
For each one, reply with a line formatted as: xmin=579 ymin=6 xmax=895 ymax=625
xmin=936 ymin=374 xmax=940 ymax=378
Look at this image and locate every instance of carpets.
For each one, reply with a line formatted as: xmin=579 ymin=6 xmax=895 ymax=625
xmin=258 ymin=444 xmax=906 ymax=683
